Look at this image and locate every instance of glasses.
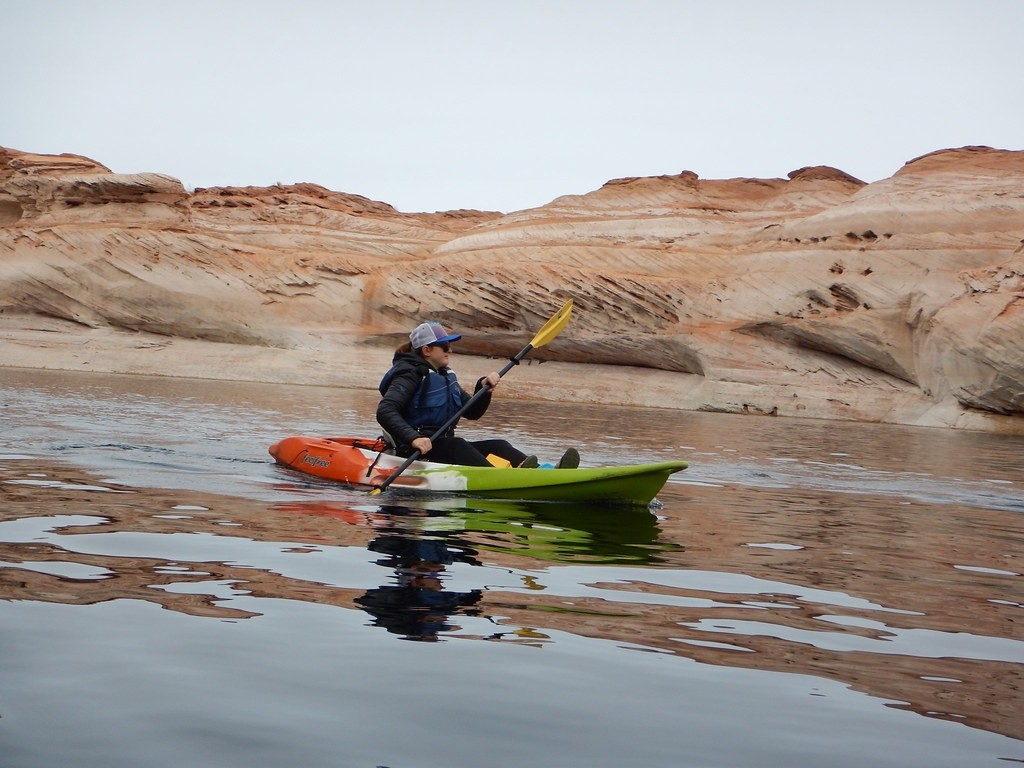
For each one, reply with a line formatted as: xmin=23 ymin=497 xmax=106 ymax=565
xmin=427 ymin=341 xmax=450 ymax=353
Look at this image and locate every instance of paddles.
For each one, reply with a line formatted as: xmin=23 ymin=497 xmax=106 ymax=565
xmin=363 ymin=298 xmax=573 ymax=496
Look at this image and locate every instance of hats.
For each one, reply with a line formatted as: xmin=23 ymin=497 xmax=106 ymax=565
xmin=408 ymin=322 xmax=462 ymax=350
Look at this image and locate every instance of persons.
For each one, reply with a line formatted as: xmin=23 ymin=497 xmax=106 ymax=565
xmin=375 ymin=321 xmax=580 ymax=469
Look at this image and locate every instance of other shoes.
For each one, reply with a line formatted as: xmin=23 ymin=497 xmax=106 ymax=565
xmin=516 ymin=454 xmax=538 ymax=468
xmin=553 ymin=447 xmax=580 ymax=469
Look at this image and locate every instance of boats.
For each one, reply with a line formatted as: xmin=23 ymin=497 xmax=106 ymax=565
xmin=266 ymin=476 xmax=672 ymax=564
xmin=267 ymin=434 xmax=689 ymax=504
